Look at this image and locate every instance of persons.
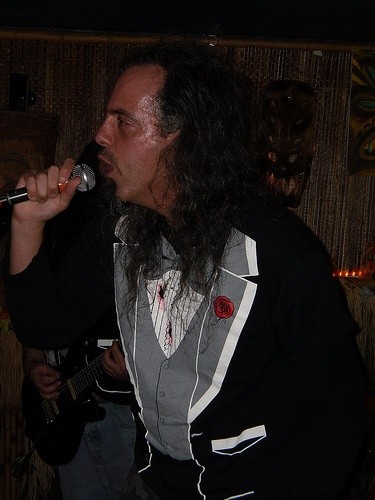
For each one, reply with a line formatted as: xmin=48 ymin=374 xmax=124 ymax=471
xmin=24 ymin=340 xmax=160 ymax=500
xmin=9 ymin=47 xmax=375 ymax=500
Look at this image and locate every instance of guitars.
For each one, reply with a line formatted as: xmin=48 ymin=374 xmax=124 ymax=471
xmin=21 ymin=340 xmax=124 ymax=467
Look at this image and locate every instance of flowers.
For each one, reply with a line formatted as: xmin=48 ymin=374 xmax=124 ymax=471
xmin=214 ymin=296 xmax=234 ymax=319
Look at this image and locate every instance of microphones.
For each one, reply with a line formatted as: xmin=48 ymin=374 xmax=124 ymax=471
xmin=0 ymin=164 xmax=96 ymax=208
